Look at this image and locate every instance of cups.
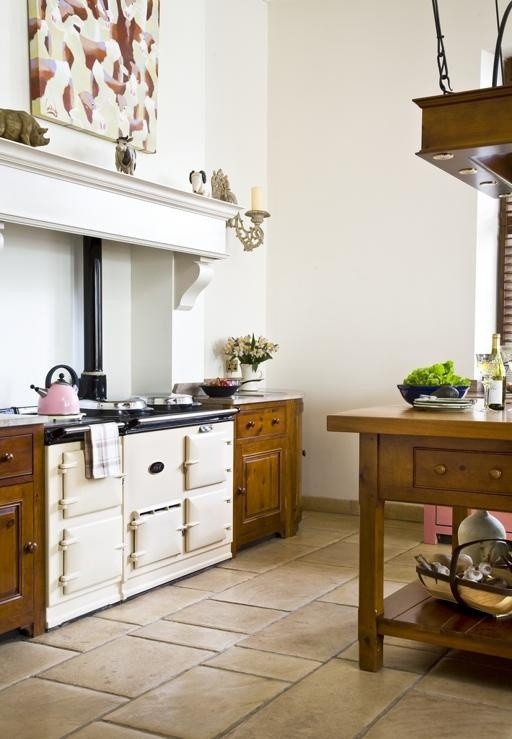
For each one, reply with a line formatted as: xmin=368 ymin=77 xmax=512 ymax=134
xmin=499 ymin=345 xmax=512 ymax=392
xmin=458 ymin=509 xmax=506 ymax=565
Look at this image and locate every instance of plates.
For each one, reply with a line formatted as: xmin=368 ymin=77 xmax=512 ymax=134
xmin=82 ymin=392 xmax=201 ymax=418
xmin=412 ymin=398 xmax=475 ymax=411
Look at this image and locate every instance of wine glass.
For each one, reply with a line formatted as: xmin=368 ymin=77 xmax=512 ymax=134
xmin=475 ymin=354 xmax=498 ymax=413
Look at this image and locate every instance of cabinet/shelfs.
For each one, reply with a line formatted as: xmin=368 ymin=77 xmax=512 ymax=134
xmin=422 ymin=504 xmax=512 ymax=545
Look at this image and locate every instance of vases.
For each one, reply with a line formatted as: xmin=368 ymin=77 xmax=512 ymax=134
xmin=240 ymin=364 xmax=263 ymax=391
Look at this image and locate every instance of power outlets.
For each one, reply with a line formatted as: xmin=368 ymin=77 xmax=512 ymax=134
xmin=226 ymin=360 xmax=238 ymax=372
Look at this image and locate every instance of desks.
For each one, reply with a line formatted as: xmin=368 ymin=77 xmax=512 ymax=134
xmin=320 ymin=396 xmax=512 ymax=674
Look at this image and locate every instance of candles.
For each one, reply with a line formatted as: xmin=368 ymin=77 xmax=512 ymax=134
xmin=250 ymin=186 xmax=266 ymax=210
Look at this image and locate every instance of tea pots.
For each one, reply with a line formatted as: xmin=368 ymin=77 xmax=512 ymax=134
xmin=30 ymin=364 xmax=82 ymax=416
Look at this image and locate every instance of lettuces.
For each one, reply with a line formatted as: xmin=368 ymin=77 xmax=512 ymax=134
xmin=403 ymin=361 xmax=470 ymax=387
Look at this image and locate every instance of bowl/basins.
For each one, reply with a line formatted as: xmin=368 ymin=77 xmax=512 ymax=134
xmin=397 ymin=384 xmax=469 ymax=407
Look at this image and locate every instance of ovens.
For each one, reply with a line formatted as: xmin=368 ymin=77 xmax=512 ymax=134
xmin=45 ymin=405 xmax=239 ymax=630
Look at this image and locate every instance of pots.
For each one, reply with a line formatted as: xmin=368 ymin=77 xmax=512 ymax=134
xmin=199 ymin=378 xmax=262 ymax=398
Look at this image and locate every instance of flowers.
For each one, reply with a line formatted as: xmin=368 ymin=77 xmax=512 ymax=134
xmin=223 ymin=334 xmax=280 ymax=373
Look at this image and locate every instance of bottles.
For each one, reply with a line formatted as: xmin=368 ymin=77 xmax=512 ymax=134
xmin=415 ymin=551 xmax=437 ymax=571
xmin=488 ymin=331 xmax=504 ymax=412
